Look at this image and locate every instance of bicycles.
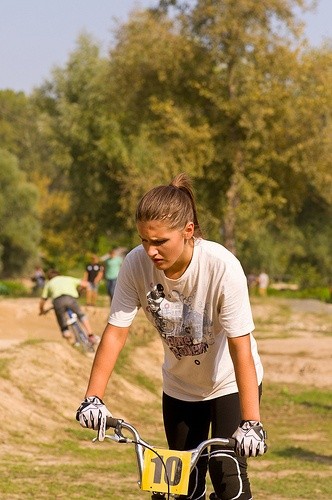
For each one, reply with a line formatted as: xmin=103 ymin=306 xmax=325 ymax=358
xmin=40 ymin=287 xmax=95 ymax=355
xmin=90 ymin=415 xmax=268 ymax=500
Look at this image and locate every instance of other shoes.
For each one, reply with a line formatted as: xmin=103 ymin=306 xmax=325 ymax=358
xmin=90 ymin=335 xmax=102 ymax=345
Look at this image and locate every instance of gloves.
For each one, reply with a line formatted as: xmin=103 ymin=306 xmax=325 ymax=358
xmin=231 ymin=420 xmax=267 ymax=459
xmin=75 ymin=395 xmax=114 ymax=442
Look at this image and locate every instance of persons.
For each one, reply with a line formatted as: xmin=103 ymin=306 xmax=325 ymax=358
xmin=77 ymin=173 xmax=265 ymax=500
xmin=103 ymin=248 xmax=123 ymax=299
xmin=245 ymin=270 xmax=269 ymax=298
xmin=83 ymin=253 xmax=104 ymax=306
xmin=40 ymin=269 xmax=101 ymax=344
xmin=30 ymin=266 xmax=47 ymax=296
